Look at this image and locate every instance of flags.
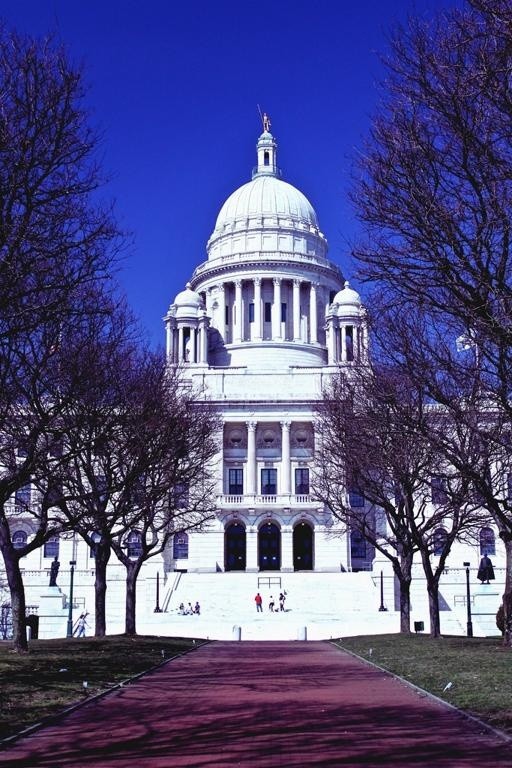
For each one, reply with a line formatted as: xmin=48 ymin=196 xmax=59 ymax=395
xmin=456 ymin=325 xmax=477 ymax=352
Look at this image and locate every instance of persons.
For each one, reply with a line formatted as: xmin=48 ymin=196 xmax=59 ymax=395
xmin=262 ymin=113 xmax=272 ymax=132
xmin=255 ymin=593 xmax=263 ymax=612
xmin=269 ymin=595 xmax=274 ymax=610
xmin=48 ymin=557 xmax=60 ymax=587
xmin=477 ymin=552 xmax=495 ymax=584
xmin=77 ymin=615 xmax=92 ymax=637
xmin=279 ymin=592 xmax=286 ymax=612
xmin=177 ymin=602 xmax=200 ymax=616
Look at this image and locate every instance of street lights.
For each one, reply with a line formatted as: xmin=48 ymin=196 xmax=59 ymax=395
xmin=463 ymin=562 xmax=473 ymax=635
xmin=68 ymin=561 xmax=76 ymax=637
